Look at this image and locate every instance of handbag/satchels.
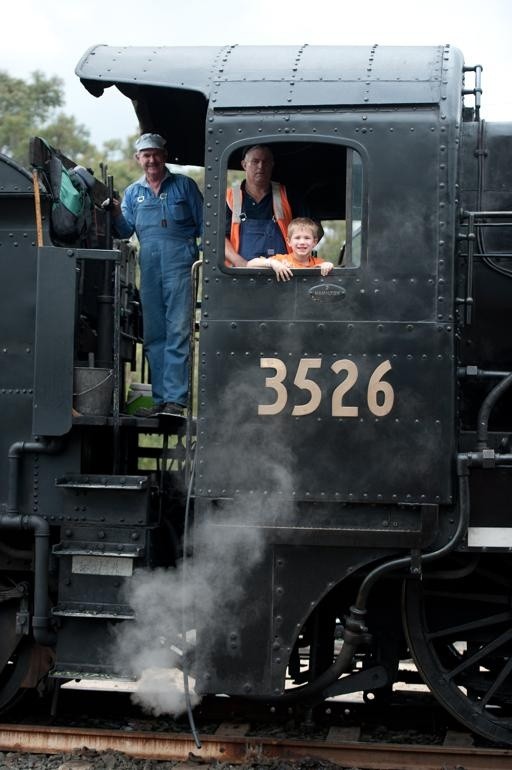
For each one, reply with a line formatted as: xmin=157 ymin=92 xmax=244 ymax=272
xmin=37 ymin=135 xmax=93 ymax=246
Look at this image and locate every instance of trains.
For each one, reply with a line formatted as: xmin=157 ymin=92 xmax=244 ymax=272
xmin=0 ymin=42 xmax=512 ymax=747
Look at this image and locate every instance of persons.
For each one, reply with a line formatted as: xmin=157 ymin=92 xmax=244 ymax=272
xmin=221 ymin=142 xmax=295 ymax=269
xmin=244 ymin=213 xmax=335 ymax=283
xmin=101 ymin=130 xmax=203 ymax=421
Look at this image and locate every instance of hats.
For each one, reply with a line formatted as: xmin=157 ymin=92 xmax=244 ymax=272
xmin=134 ymin=132 xmax=166 ymax=151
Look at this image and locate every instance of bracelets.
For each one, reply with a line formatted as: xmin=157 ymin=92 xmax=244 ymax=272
xmin=265 ymin=257 xmax=272 ymax=267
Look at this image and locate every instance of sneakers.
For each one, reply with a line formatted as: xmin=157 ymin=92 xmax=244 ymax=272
xmin=157 ymin=402 xmax=184 ymax=417
xmin=133 ymin=405 xmax=162 ymax=417
